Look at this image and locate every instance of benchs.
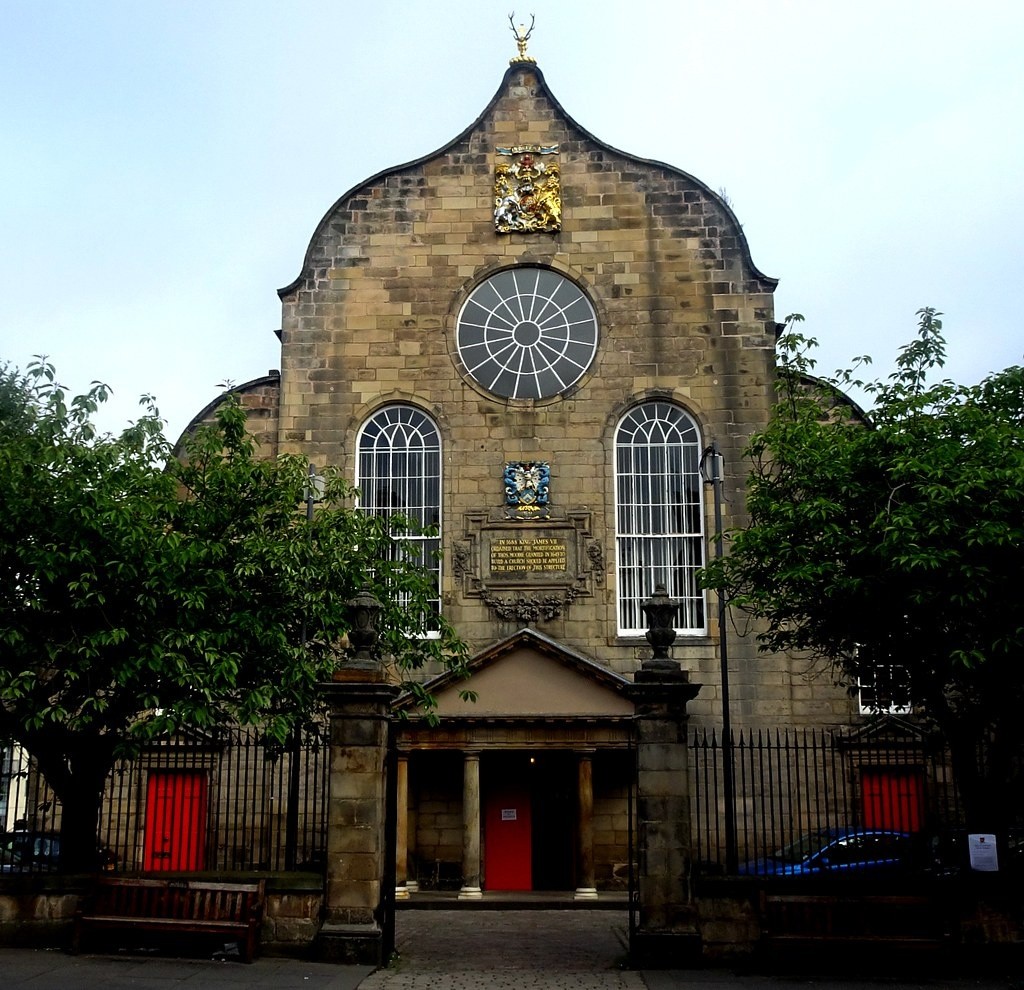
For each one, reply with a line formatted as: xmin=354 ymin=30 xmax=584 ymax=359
xmin=756 ymin=889 xmax=951 ymax=976
xmin=69 ymin=874 xmax=266 ymax=962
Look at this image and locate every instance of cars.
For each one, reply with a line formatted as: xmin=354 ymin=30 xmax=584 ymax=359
xmin=0 ymin=832 xmax=124 ymax=873
xmin=686 ymin=826 xmax=925 ymax=901
